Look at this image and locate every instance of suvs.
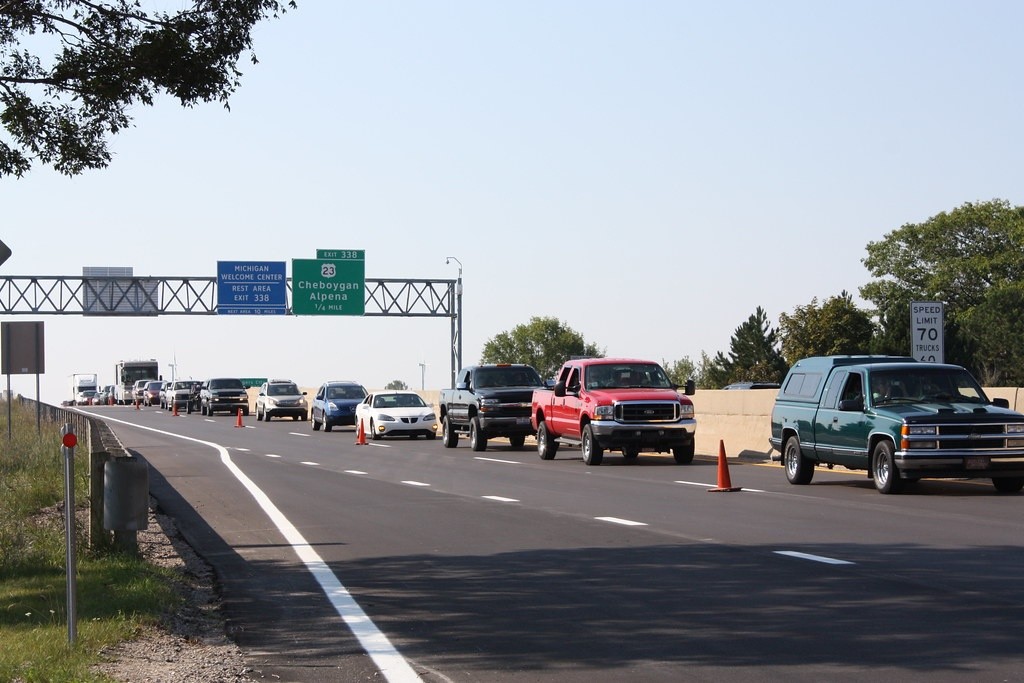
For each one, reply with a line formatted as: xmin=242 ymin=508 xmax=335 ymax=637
xmin=255 ymin=380 xmax=308 ymax=422
xmin=199 ymin=378 xmax=250 ymax=416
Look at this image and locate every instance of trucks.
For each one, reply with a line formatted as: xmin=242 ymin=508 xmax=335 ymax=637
xmin=71 ymin=373 xmax=98 ymax=406
xmin=113 ymin=358 xmax=161 ymax=405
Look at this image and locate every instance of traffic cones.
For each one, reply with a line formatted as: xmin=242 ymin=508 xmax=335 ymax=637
xmin=234 ymin=408 xmax=246 ymax=427
xmin=707 ymin=439 xmax=744 ymax=492
xmin=108 ymin=396 xmax=113 ymax=405
xmin=134 ymin=398 xmax=142 ymax=411
xmin=353 ymin=417 xmax=369 ymax=445
xmin=172 ymin=401 xmax=180 ymax=416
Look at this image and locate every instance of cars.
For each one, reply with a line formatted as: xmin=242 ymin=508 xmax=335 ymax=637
xmin=142 ymin=380 xmax=164 ymax=406
xmin=311 ymin=381 xmax=369 ymax=433
xmin=355 ymin=390 xmax=438 ymax=440
xmin=159 ymin=381 xmax=172 ymax=410
xmin=131 ymin=380 xmax=152 ymax=406
xmin=91 ymin=385 xmax=117 ymax=405
xmin=724 ymin=380 xmax=781 ymax=392
xmin=166 ymin=381 xmax=202 ymax=411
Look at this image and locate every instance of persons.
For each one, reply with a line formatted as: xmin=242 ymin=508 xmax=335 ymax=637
xmin=873 ymin=381 xmax=909 ymax=403
xmin=328 ymin=389 xmax=337 ymax=398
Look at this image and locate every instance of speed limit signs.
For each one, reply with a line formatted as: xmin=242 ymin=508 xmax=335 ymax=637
xmin=911 ymin=301 xmax=944 ymax=364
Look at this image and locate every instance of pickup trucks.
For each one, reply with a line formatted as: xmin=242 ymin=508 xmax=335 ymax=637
xmin=769 ymin=355 xmax=1024 ymax=494
xmin=531 ymin=357 xmax=696 ymax=467
xmin=438 ymin=363 xmax=550 ymax=452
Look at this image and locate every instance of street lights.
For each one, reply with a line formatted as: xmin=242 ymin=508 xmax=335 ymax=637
xmin=445 ymin=256 xmax=463 ymax=375
xmin=419 ymin=362 xmax=425 ymax=390
